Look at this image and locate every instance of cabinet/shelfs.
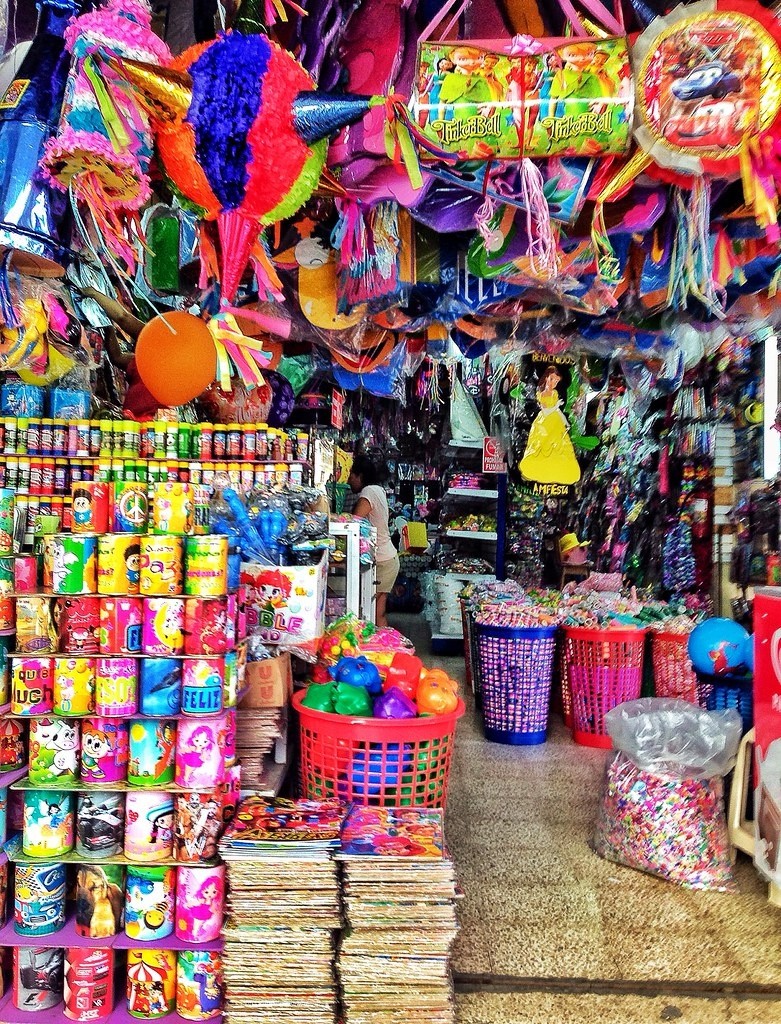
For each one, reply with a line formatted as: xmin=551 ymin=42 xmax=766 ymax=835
xmin=325 ymin=521 xmax=377 ymax=624
xmin=0 ymin=583 xmax=255 ymax=1024
xmin=428 ymin=488 xmax=499 ymax=656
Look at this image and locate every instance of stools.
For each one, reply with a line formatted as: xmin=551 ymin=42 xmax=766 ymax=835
xmin=726 ymin=726 xmax=781 ymax=907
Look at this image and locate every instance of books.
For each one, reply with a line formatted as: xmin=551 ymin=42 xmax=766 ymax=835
xmin=217 ymin=796 xmax=464 ymax=1024
xmin=233 ymin=709 xmax=288 ymax=788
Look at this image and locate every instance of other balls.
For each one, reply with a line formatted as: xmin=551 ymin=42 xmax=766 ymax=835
xmin=687 ymin=618 xmax=756 ymax=730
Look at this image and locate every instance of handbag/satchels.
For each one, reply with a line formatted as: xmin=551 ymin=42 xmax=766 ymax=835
xmin=415 ymin=0 xmax=636 ymax=163
xmin=401 ymin=0 xmax=602 ymax=228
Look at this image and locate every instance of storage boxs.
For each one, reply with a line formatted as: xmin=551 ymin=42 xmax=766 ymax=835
xmin=236 ymin=651 xmax=294 ymax=708
xmin=239 ymin=547 xmax=329 ymax=641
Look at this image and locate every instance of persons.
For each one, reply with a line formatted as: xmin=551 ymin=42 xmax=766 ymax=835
xmin=347 ymin=454 xmax=401 ymax=630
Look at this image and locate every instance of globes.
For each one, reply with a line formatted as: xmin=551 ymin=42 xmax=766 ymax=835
xmin=688 ymin=617 xmax=754 ymax=678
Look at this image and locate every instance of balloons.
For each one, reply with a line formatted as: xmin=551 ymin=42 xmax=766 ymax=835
xmin=136 ymin=310 xmax=218 ymax=408
